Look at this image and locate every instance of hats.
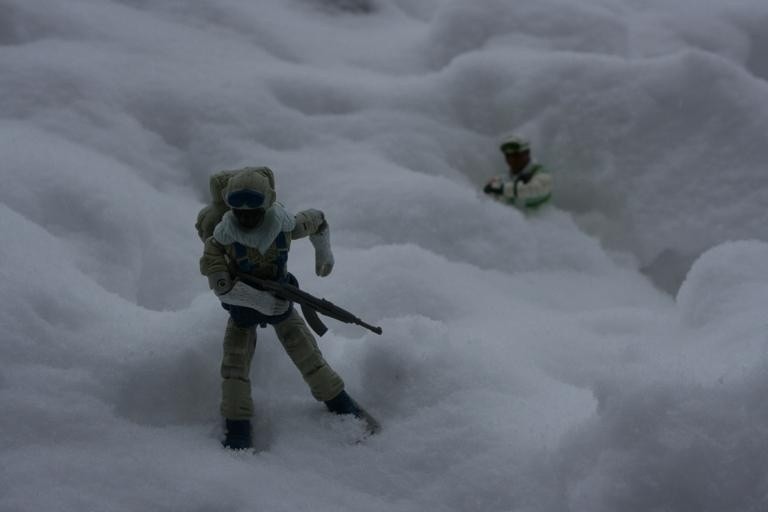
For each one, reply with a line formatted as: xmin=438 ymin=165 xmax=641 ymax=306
xmin=500 ymin=133 xmax=531 ymax=156
